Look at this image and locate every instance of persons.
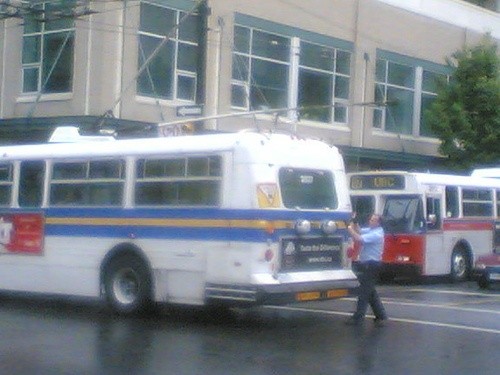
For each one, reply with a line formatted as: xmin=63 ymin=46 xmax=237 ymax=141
xmin=0 ymin=213 xmax=9 ymax=253
xmin=345 ymin=212 xmax=388 ymax=324
xmin=58 ymin=187 xmax=83 ymax=205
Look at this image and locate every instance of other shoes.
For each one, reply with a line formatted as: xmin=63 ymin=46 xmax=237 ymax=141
xmin=345 ymin=317 xmax=363 ymax=324
xmin=373 ymin=317 xmax=390 ymax=327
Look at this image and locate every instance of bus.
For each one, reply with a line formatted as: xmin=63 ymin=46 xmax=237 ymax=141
xmin=0 ymin=132 xmax=362 ymax=320
xmin=346 ymin=165 xmax=499 ymax=284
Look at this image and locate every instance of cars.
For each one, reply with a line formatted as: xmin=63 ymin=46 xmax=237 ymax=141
xmin=472 ymin=252 xmax=500 ymax=290
